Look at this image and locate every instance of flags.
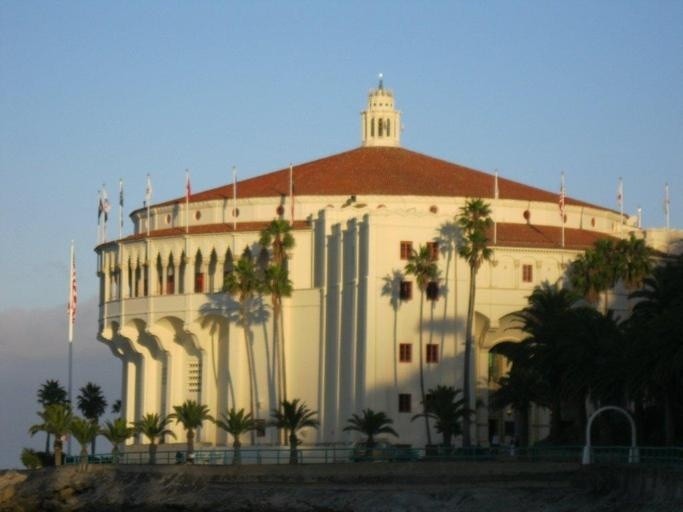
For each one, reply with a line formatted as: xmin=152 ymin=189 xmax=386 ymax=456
xmin=95 ymin=176 xmax=296 ymax=227
xmin=65 ymin=244 xmax=77 ymax=325
xmin=558 ymin=183 xmax=670 ymax=225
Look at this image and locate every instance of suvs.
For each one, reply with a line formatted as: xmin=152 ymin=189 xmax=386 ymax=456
xmin=348 ymin=438 xmax=413 ymax=462
